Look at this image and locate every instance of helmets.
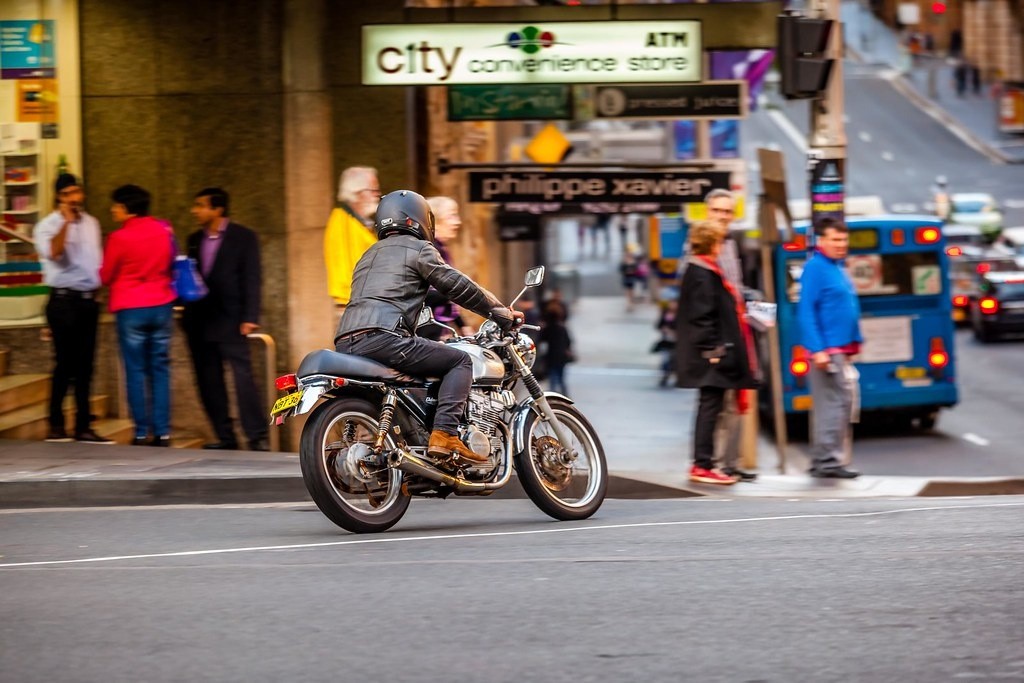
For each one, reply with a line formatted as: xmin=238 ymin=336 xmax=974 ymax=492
xmin=375 ymin=189 xmax=436 ymax=244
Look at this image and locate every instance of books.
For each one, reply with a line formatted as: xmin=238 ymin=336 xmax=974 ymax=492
xmin=5 ymin=162 xmax=37 ymax=244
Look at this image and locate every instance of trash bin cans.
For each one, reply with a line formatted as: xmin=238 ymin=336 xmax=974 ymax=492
xmin=552 ymin=264 xmax=579 ymax=304
xmin=997 ymin=80 xmax=1024 ymax=134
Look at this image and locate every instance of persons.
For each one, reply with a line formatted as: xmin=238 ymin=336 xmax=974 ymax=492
xmin=518 ymin=281 xmax=578 ymax=397
xmin=323 ymin=164 xmax=380 ymax=320
xmin=31 ymin=172 xmax=119 ymax=445
xmin=618 ymin=249 xmax=653 ymax=313
xmin=177 ymin=185 xmax=273 ymax=452
xmin=931 ymin=176 xmax=958 ymax=221
xmin=333 ymin=185 xmax=527 ymax=465
xmin=666 ymin=187 xmax=754 ymax=485
xmin=798 ymin=206 xmax=862 ymax=479
xmin=416 ymin=191 xmax=475 ymax=341
xmin=906 ymin=24 xmax=984 ymax=97
xmin=97 ymin=184 xmax=176 ymax=449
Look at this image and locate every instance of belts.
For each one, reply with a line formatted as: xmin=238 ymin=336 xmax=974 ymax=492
xmin=336 ymin=328 xmax=375 ymax=344
xmin=52 ymin=287 xmax=98 ymax=299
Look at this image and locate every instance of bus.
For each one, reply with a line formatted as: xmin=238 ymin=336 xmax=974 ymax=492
xmin=730 ymin=215 xmax=959 ymax=429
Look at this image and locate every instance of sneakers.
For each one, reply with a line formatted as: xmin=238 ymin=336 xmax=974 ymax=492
xmin=427 ymin=430 xmax=489 ymax=466
xmin=690 ymin=465 xmax=738 ymax=484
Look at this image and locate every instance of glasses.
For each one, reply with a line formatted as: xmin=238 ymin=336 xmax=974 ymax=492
xmin=357 ymin=186 xmax=384 ymax=198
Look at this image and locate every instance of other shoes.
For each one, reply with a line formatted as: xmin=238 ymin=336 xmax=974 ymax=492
xmin=719 ymin=465 xmax=757 ymax=482
xmin=250 ymin=437 xmax=271 ymax=451
xmin=808 ymin=463 xmax=859 ymax=481
xmin=73 ymin=428 xmax=117 ymax=444
xmin=132 ymin=435 xmax=147 ymax=444
xmin=43 ymin=430 xmax=75 ymax=443
xmin=148 ymin=433 xmax=171 ymax=447
xmin=202 ymin=438 xmax=240 ymax=450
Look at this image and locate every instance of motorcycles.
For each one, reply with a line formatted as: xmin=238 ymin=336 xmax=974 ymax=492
xmin=269 ymin=264 xmax=608 ymax=535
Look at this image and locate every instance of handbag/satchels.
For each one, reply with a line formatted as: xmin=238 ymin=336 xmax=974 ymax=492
xmin=169 ymin=253 xmax=210 ymax=300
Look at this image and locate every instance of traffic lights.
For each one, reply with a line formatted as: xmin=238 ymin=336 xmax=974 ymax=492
xmin=775 ymin=8 xmax=836 ymax=99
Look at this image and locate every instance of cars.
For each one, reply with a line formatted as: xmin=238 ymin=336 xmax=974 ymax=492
xmin=991 ymin=226 xmax=1024 ymax=258
xmin=948 ymin=192 xmax=1004 ymax=240
xmin=947 ymin=271 xmax=978 ymax=323
xmin=975 ymin=257 xmax=1024 ymax=282
xmin=970 ymin=270 xmax=1024 ymax=336
xmin=941 ymin=224 xmax=984 ymax=257
